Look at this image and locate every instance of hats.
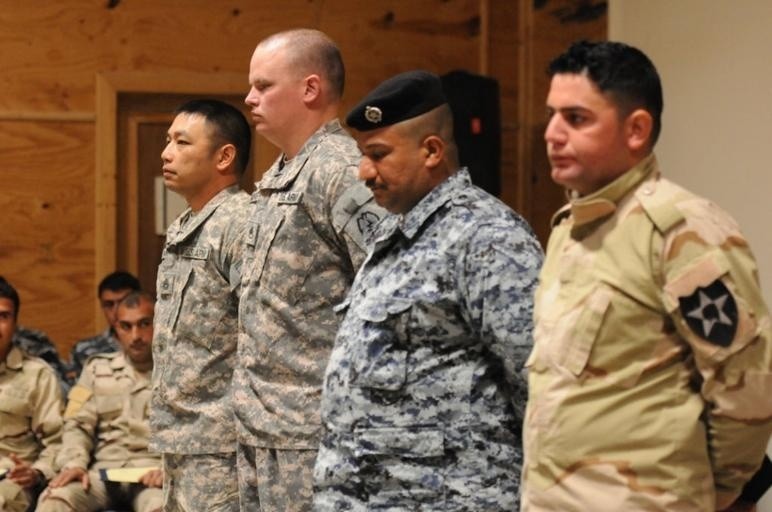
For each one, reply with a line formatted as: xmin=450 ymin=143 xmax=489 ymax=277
xmin=346 ymin=71 xmax=447 ymax=132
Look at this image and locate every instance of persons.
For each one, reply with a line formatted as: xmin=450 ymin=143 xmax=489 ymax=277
xmin=1 ymin=281 xmax=66 ymax=512
xmin=312 ymin=71 xmax=542 ymax=512
xmin=235 ymin=28 xmax=389 ymax=511
xmin=522 ymin=40 xmax=771 ymax=511
xmin=69 ymin=271 xmax=143 ymax=387
xmin=36 ymin=291 xmax=165 ymax=510
xmin=148 ymin=100 xmax=261 ymax=512
